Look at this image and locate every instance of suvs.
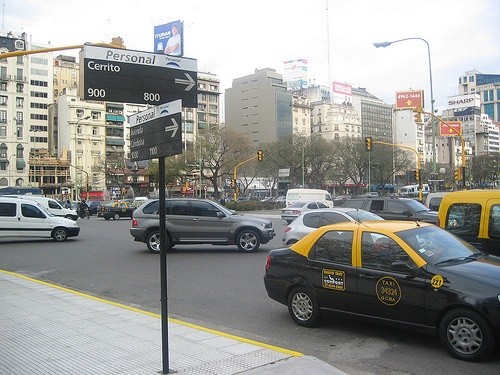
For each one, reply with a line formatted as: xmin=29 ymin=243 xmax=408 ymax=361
xmin=130 ymin=198 xmax=276 ymax=253
xmin=335 ymin=195 xmax=462 ymax=230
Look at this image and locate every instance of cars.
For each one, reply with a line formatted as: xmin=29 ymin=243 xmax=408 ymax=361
xmin=264 ymin=220 xmax=500 ymax=363
xmin=281 ymin=199 xmax=330 ymax=225
xmin=218 ymin=196 xmax=248 ymax=203
xmin=261 ymin=195 xmax=285 ymax=203
xmin=335 ymin=194 xmax=352 ymax=200
xmin=282 ymin=206 xmax=426 ymax=249
xmin=54 ymin=197 xmax=149 ymax=219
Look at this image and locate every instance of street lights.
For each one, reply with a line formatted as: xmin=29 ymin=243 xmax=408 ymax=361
xmin=372 ymin=38 xmax=438 ymax=195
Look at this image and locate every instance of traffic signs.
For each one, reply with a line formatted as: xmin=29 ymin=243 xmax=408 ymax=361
xmin=78 ymin=44 xmax=199 ymax=110
xmin=129 ymin=98 xmax=183 ymax=162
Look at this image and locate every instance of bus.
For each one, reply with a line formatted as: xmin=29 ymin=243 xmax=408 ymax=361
xmin=399 ymin=184 xmax=431 ymax=198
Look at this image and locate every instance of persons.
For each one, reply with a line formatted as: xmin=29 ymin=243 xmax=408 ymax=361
xmin=79 ymin=200 xmax=90 ymax=218
xmin=65 ymin=200 xmax=72 ymax=210
xmin=164 ymin=24 xmax=181 ymax=56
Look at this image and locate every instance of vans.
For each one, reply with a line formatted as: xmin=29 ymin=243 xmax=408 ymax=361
xmin=436 ymin=187 xmax=500 ymax=257
xmin=0 ymin=186 xmax=79 ymax=221
xmin=285 ymin=189 xmax=335 ymax=208
xmin=0 ymin=197 xmax=80 ymax=242
xmin=425 ymin=191 xmax=466 ymax=218
xmin=364 ymin=191 xmax=379 ymax=196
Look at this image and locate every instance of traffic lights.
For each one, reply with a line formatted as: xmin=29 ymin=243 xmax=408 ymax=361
xmin=365 ymin=137 xmax=372 ymax=151
xmin=413 ymin=107 xmax=422 ymax=122
xmin=454 ymin=167 xmax=462 ymax=181
xmin=257 ymin=149 xmax=263 ymax=162
xmin=414 ymin=170 xmax=419 ymax=182
xmin=231 ymin=179 xmax=235 ymax=188
